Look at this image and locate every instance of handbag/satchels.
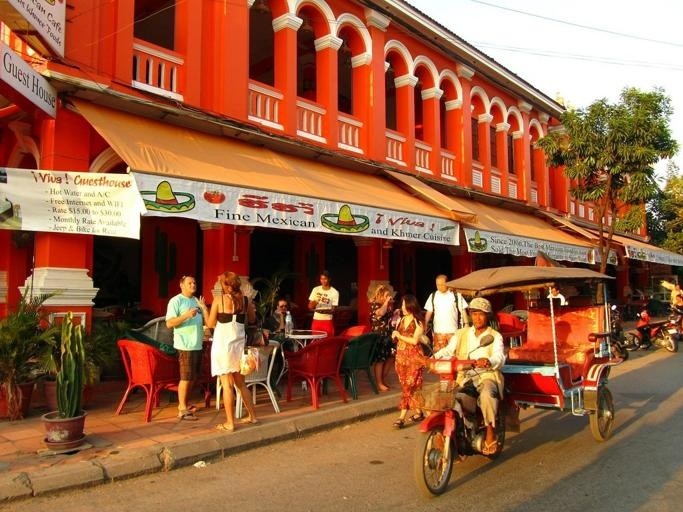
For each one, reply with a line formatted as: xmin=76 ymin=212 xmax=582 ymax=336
xmin=419 ymin=335 xmax=432 ymax=357
xmin=240 ymin=345 xmax=261 ymax=376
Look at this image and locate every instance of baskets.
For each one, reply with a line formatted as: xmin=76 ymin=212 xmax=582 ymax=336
xmin=416 ymin=381 xmax=460 ymax=411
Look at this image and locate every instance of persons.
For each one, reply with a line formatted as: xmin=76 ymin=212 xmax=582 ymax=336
xmin=165 ymin=275 xmax=205 ymax=418
xmin=193 ymin=270 xmax=260 ymax=431
xmin=368 ymin=283 xmax=395 ymax=392
xmin=422 ymin=274 xmax=470 ymax=353
xmin=546 ymin=287 xmax=568 ymax=308
xmin=306 ymin=269 xmax=340 ymax=338
xmin=390 ymin=294 xmax=426 ymax=429
xmin=426 ymin=297 xmax=506 ymax=456
xmin=631 ymin=279 xmax=683 ymax=350
xmin=267 ymin=296 xmax=295 ymax=381
xmin=209 ymin=301 xmax=258 ymax=346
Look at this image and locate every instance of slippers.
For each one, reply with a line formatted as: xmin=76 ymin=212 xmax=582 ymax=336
xmin=187 ymin=405 xmax=200 ymax=412
xmin=238 ymin=416 xmax=259 ymax=426
xmin=177 ymin=413 xmax=199 ymax=421
xmin=215 ymin=423 xmax=235 ymax=433
xmin=482 ymin=434 xmax=499 ymax=455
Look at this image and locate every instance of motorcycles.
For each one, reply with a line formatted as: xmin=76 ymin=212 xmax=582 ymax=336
xmin=414 ymin=265 xmax=615 ymax=500
xmin=606 ymin=305 xmax=677 ymax=365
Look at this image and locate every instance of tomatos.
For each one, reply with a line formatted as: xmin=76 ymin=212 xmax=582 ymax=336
xmin=203 ymin=190 xmax=225 ymax=204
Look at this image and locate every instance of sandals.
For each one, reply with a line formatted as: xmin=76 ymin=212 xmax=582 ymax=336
xmin=392 ymin=417 xmax=406 ymax=427
xmin=408 ymin=413 xmax=424 ymax=422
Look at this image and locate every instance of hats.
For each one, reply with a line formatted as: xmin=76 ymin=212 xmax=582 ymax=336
xmin=464 ymin=297 xmax=493 ymax=317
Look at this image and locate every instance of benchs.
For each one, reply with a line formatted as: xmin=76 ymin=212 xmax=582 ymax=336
xmin=508 ymin=304 xmax=604 ymax=379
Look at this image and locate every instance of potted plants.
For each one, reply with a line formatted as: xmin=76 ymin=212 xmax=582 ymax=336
xmin=0 ymin=286 xmax=58 ymax=420
xmin=41 ymin=311 xmax=88 ymax=450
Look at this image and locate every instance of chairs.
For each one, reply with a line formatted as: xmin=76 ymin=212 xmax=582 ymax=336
xmin=497 ymin=312 xmax=525 ymax=348
xmin=115 ymin=314 xmax=383 ymax=424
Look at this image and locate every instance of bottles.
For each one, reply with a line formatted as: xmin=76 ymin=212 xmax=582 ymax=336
xmin=285 ymin=311 xmax=293 ymax=335
xmin=599 ymin=338 xmax=606 ymax=357
